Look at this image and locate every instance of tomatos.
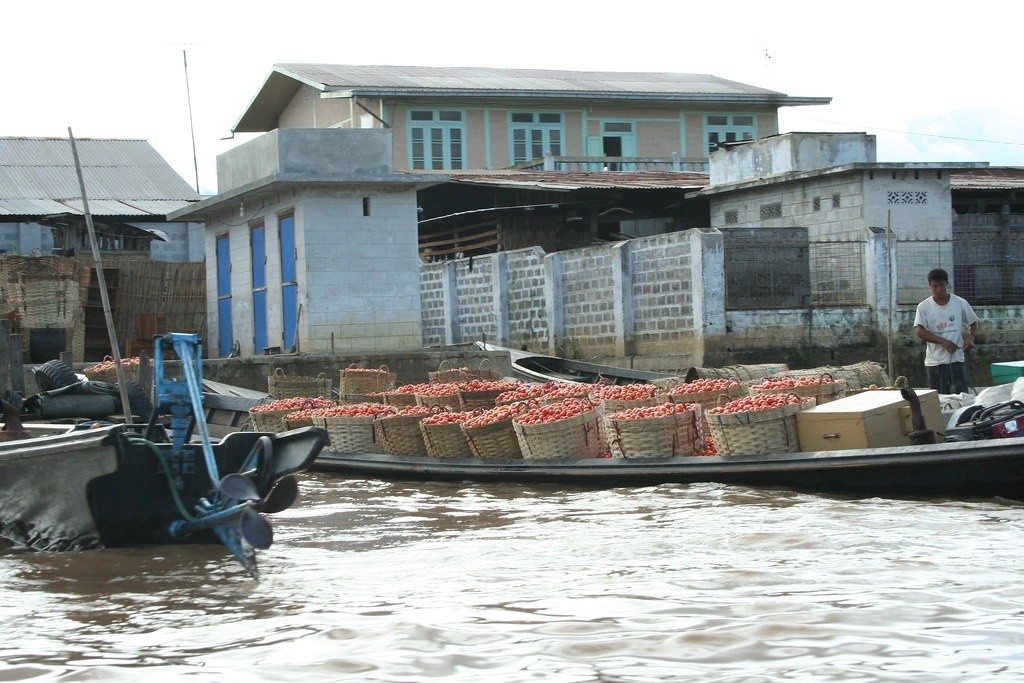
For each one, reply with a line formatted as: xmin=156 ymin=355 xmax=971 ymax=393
xmin=249 ymin=368 xmax=878 ymax=457
xmin=87 ymin=357 xmax=139 ymax=372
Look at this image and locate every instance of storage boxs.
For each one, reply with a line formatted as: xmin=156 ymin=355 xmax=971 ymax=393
xmin=138 ymin=312 xmax=168 ymax=342
xmin=796 ymin=390 xmax=946 ymax=453
xmin=0 ymin=246 xmax=82 ymax=330
xmin=249 ymin=361 xmax=890 ymax=460
xmin=125 ymin=339 xmax=155 ymax=360
xmin=83 ymin=352 xmax=140 ymax=384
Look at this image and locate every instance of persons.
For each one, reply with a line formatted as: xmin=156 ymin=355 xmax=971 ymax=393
xmin=913 ymin=268 xmax=981 ymax=394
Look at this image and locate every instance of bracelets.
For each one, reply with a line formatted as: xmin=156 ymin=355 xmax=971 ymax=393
xmin=968 ymin=332 xmax=977 ymax=336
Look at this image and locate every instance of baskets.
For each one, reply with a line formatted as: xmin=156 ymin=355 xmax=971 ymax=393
xmin=85 ymin=356 xmax=154 ymax=405
xmin=245 ymin=359 xmax=890 ymax=465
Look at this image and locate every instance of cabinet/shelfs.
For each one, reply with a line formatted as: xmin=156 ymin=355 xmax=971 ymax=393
xmin=79 ymin=266 xmax=119 ymax=361
